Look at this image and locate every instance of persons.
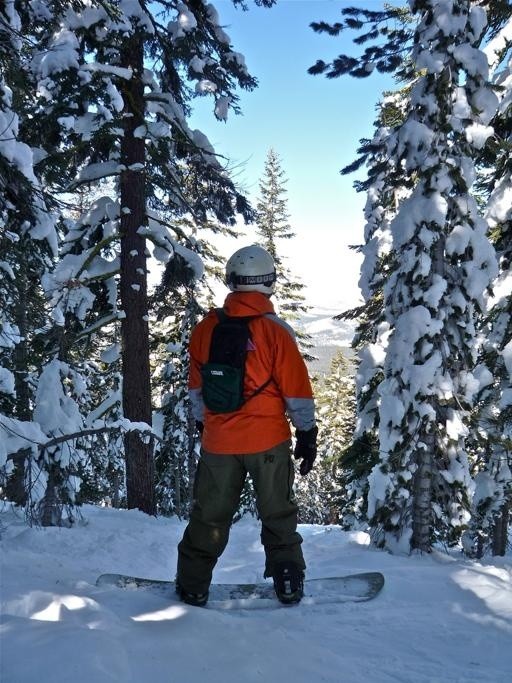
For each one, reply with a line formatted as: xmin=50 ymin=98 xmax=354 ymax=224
xmin=174 ymin=243 xmax=317 ymax=608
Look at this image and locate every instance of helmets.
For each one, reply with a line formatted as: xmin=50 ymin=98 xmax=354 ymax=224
xmin=226 ymin=245 xmax=276 ymax=295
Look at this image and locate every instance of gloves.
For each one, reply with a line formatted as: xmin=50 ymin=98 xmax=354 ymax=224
xmin=294 ymin=425 xmax=318 ymax=475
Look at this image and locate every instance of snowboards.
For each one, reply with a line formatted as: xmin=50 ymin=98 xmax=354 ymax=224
xmin=97 ymin=571 xmax=384 ymax=612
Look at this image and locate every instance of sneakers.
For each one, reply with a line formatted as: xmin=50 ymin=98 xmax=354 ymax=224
xmin=273 ymin=561 xmax=305 ymax=606
xmin=176 ymin=574 xmax=208 ymax=606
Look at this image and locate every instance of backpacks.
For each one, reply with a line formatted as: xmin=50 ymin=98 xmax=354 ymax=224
xmin=200 ymin=308 xmax=274 ymax=413
